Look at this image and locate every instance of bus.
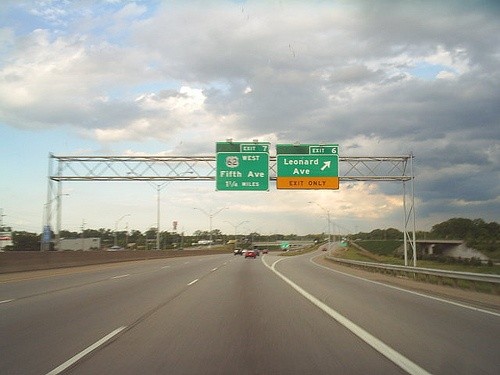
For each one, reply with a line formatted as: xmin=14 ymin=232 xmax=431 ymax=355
xmin=0 ymin=225 xmax=15 ymax=251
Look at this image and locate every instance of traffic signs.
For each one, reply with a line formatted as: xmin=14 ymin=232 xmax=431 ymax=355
xmin=275 ymin=153 xmax=340 ymax=190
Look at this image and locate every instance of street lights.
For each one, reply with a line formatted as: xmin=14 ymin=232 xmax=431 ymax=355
xmin=114 ymin=213 xmax=132 ymax=246
xmin=193 ymin=207 xmax=229 ymax=248
xmin=42 ymin=194 xmax=71 ymax=232
xmin=219 ymin=220 xmax=250 ymax=248
xmin=308 ymin=201 xmax=349 ymax=258
xmin=126 ymin=170 xmax=194 ymax=251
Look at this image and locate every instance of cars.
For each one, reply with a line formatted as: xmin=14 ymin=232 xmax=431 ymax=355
xmin=322 ymin=247 xmax=327 ymax=251
xmin=233 ymin=247 xmax=268 ymax=259
